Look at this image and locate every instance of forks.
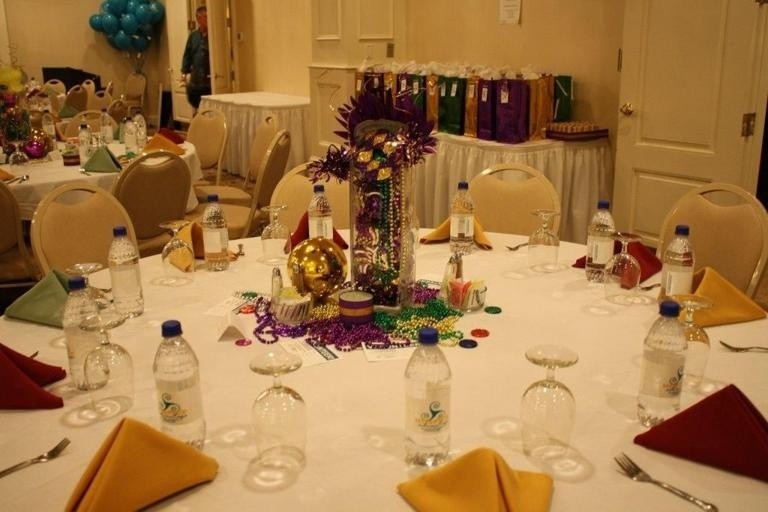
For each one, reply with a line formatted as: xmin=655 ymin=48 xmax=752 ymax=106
xmin=719 ymin=340 xmax=767 ymax=354
xmin=0 ymin=435 xmax=73 ymax=480
xmin=612 ymin=451 xmax=717 ymax=512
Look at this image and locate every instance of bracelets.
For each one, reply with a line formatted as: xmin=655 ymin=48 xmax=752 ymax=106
xmin=181 ymin=73 xmax=186 ymax=77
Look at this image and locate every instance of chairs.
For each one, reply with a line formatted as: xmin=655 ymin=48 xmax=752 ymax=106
xmin=466 ymin=161 xmax=562 ymax=239
xmin=30 ymin=178 xmax=140 ymax=278
xmin=1 ymin=183 xmax=45 ymax=311
xmin=266 ymin=159 xmax=350 ymax=238
xmin=193 ymin=110 xmax=281 ymax=207
xmin=114 ymin=71 xmax=147 ymax=131
xmin=179 ymin=128 xmax=294 ymax=241
xmin=0 ymin=69 xmax=127 ymax=147
xmin=656 ymin=181 xmax=767 ymax=302
xmin=182 ymin=107 xmax=228 ymax=186
xmin=109 ymin=149 xmax=192 ymax=260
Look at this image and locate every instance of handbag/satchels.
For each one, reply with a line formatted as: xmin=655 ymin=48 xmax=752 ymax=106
xmin=355 ymin=57 xmax=574 ymax=145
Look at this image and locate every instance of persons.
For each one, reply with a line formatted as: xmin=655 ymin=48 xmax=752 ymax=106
xmin=179 ymin=3 xmax=211 ymax=117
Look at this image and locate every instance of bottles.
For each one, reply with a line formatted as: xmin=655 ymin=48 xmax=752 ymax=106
xmin=23 ymin=72 xmax=149 ymax=171
xmin=583 ymin=199 xmax=616 ymax=282
xmin=58 ymin=278 xmax=112 ymax=391
xmin=632 ymin=300 xmax=688 ymax=427
xmin=402 ymin=327 xmax=451 ymax=469
xmin=438 ymin=256 xmax=457 ymax=300
xmin=107 ymin=224 xmax=146 ymax=317
xmin=151 ymin=320 xmax=208 ymax=455
xmin=660 ymin=225 xmax=695 ymax=296
xmin=306 ymin=184 xmax=332 ymax=243
xmin=448 ymin=181 xmax=474 ymax=255
xmin=272 ymin=269 xmax=282 ymax=300
xmin=200 ymin=196 xmax=229 ymax=274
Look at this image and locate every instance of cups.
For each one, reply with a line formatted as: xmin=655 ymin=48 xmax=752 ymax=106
xmin=271 ymin=294 xmax=314 ymax=327
xmin=338 ymin=291 xmax=374 ymax=324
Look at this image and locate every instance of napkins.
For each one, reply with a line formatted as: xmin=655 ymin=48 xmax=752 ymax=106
xmin=64 ymin=418 xmax=218 ymax=512
xmin=169 ymin=219 xmax=237 ymax=272
xmin=419 ymin=206 xmax=493 ymax=251
xmin=632 ymin=382 xmax=768 ymax=485
xmin=142 ymin=132 xmax=186 ymax=157
xmin=79 ymin=145 xmax=123 ymax=173
xmin=396 ymin=446 xmax=553 ymax=512
xmin=572 ymin=231 xmax=663 ymax=286
xmin=154 ymin=128 xmax=184 ymax=144
xmin=3 ymin=268 xmax=102 ymax=330
xmin=0 ymin=341 xmax=67 ymax=410
xmin=660 ymin=266 xmax=767 ymax=328
xmin=282 ymin=210 xmax=350 ymax=256
xmin=0 ymin=167 xmax=16 ymax=184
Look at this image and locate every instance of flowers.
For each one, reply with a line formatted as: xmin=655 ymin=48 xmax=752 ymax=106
xmin=306 ymin=72 xmax=439 ymax=191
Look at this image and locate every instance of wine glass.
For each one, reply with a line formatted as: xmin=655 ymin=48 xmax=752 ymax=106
xmin=64 ymin=261 xmax=118 ymax=328
xmin=257 ymin=204 xmax=294 ymax=267
xmin=602 ymin=233 xmax=643 ymax=305
xmin=76 ymin=310 xmax=138 ymax=418
xmin=246 ymin=349 xmax=307 ymax=465
xmin=672 ymin=294 xmax=713 ymax=392
xmin=526 ymin=208 xmax=561 ymax=274
xmin=156 ymin=220 xmax=195 ymax=290
xmin=520 ymin=343 xmax=581 ymax=466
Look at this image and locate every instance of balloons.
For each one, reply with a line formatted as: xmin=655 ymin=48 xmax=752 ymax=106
xmin=89 ymin=1 xmax=165 ymax=71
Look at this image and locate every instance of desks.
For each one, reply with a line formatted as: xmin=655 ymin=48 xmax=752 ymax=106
xmin=1 ymin=136 xmax=203 ymax=242
xmin=200 ymin=91 xmax=310 ymax=183
xmin=0 ymin=229 xmax=768 ymax=512
xmin=413 ymin=128 xmax=615 ymax=245
